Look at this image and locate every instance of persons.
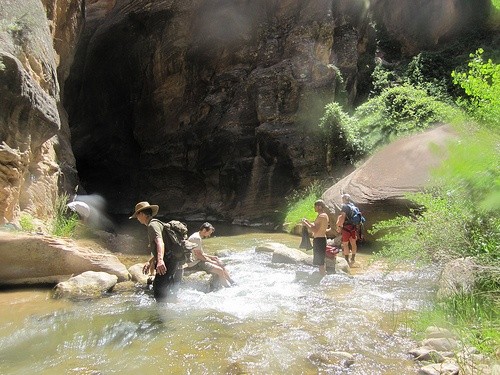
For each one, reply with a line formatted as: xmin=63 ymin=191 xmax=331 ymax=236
xmin=300 ymin=201 xmax=329 ymax=275
xmin=128 ymin=201 xmax=180 ymax=303
xmin=185 ymin=222 xmax=237 ymax=288
xmin=335 ymin=194 xmax=359 ymax=263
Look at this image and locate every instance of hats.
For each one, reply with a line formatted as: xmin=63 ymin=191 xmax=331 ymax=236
xmin=129 ymin=202 xmax=159 ymax=219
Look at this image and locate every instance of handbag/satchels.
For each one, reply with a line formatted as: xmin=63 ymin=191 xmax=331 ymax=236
xmin=325 ymin=245 xmax=339 ymax=257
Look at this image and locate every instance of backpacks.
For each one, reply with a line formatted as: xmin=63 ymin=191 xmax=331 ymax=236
xmin=345 ymin=204 xmax=365 ymax=224
xmin=150 ymin=219 xmax=198 ymax=261
